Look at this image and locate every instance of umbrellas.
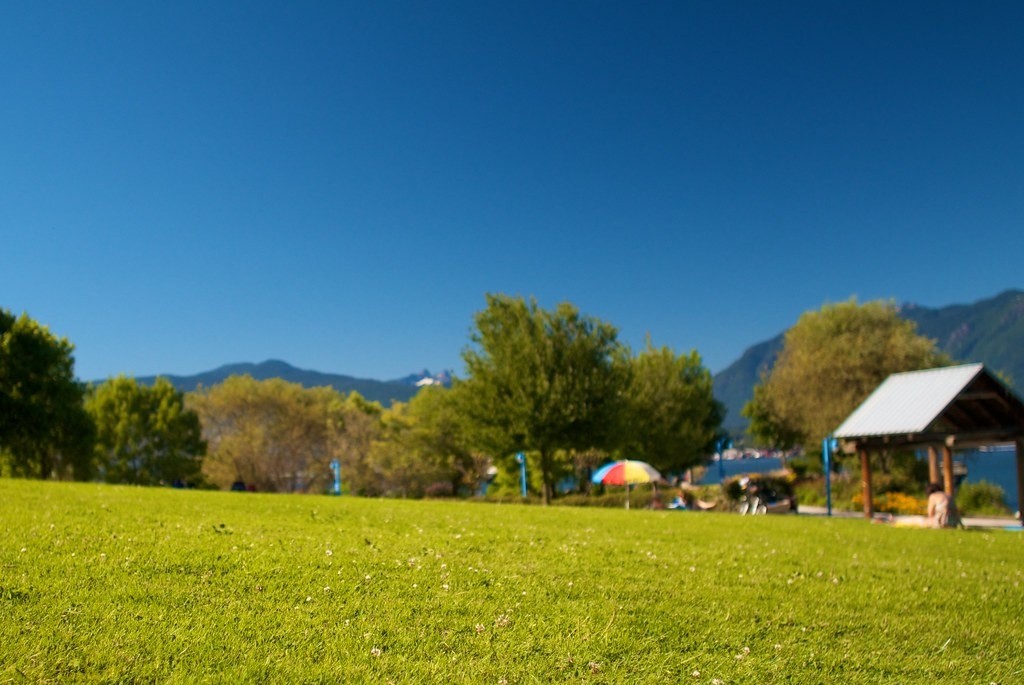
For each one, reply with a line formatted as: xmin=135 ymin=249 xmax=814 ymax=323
xmin=591 ymin=458 xmax=661 ymax=510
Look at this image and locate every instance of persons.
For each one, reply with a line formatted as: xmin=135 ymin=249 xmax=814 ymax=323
xmin=737 ymin=473 xmax=769 ymax=516
xmin=926 ymin=484 xmax=959 ymax=528
xmin=676 ymin=479 xmax=717 ymax=509
